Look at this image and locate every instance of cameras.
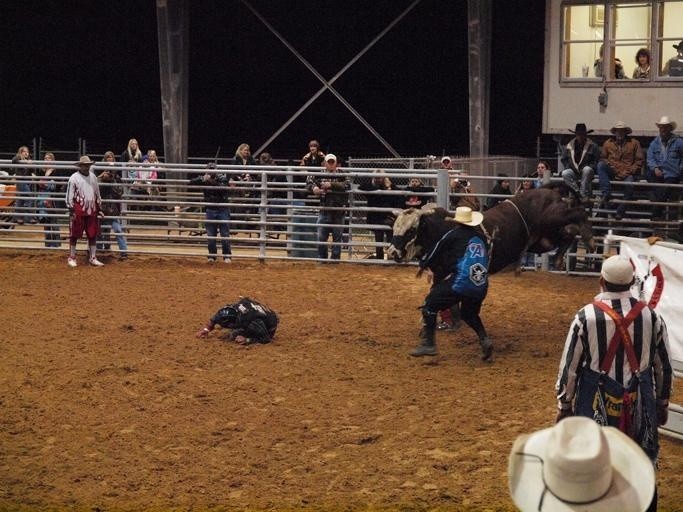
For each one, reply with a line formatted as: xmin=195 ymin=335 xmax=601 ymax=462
xmin=207 ymin=172 xmax=219 ymax=182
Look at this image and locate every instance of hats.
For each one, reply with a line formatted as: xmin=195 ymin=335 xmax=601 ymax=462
xmin=654 ymin=115 xmax=678 ymax=132
xmin=323 ymin=152 xmax=338 ymax=163
xmin=601 ymin=253 xmax=636 ymax=286
xmin=671 ymin=40 xmax=683 ymax=51
xmin=442 ymin=205 xmax=484 ymax=229
xmin=566 ymin=123 xmax=594 ymax=137
xmin=440 ymin=154 xmax=452 ymax=165
xmin=72 ymin=155 xmax=96 ymax=165
xmin=609 ymin=122 xmax=633 ymax=135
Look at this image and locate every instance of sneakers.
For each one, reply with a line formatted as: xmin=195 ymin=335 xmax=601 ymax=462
xmin=87 ymin=256 xmax=105 ymax=267
xmin=66 ymin=254 xmax=79 ymax=268
xmin=408 ymin=341 xmax=441 ymax=358
xmin=475 ymin=338 xmax=495 ymax=363
xmin=223 ymin=257 xmax=231 ymax=265
xmin=206 ymin=256 xmax=216 ymax=264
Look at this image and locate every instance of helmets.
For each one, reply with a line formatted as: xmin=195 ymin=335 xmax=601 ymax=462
xmin=210 ymin=305 xmax=238 ymax=328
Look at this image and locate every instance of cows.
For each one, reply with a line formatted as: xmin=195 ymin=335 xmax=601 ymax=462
xmin=385 ymin=181 xmax=596 ymax=339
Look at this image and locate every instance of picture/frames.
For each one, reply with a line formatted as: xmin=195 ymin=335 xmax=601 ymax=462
xmin=588 ymin=5 xmax=619 ymax=28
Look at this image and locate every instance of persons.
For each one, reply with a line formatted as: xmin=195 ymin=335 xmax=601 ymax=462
xmin=597 ymin=121 xmax=646 ymax=220
xmin=408 ymin=205 xmax=493 ymax=362
xmin=646 ymin=116 xmax=683 ymax=221
xmin=662 ymin=41 xmax=683 ymax=77
xmin=594 ymin=44 xmax=628 ymax=79
xmin=632 ymin=48 xmax=650 ymax=79
xmin=602 ymin=235 xmax=664 ymax=259
xmin=561 ymin=123 xmax=600 ymax=203
xmin=196 ymin=297 xmax=277 ymax=345
xmin=554 ymin=254 xmax=674 ymax=512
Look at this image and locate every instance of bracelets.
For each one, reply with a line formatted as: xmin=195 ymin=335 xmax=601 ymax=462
xmin=204 ymin=327 xmax=210 ymax=332
